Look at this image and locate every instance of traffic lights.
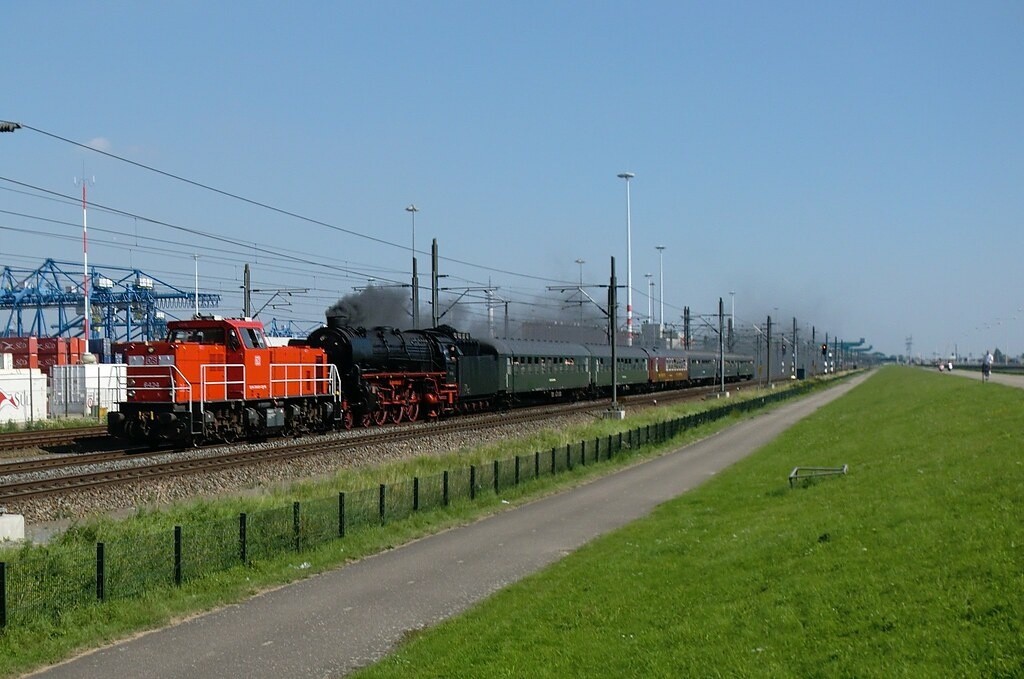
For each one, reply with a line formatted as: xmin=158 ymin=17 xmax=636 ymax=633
xmin=822 ymin=344 xmax=827 ymax=356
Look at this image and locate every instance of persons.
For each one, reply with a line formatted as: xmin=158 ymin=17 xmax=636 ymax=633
xmin=982 ymin=350 xmax=993 ymax=380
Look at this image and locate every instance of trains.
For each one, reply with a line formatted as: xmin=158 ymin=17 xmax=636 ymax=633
xmin=108 ymin=317 xmax=761 ymax=463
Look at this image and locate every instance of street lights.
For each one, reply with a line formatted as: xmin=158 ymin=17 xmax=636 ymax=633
xmin=407 ymin=203 xmax=420 ymax=331
xmin=617 ymin=171 xmax=636 ymax=345
xmin=572 ymin=260 xmax=588 ymax=325
xmin=644 ymin=270 xmax=652 ymax=324
xmin=651 ymin=282 xmax=656 ymax=323
xmin=730 ymin=290 xmax=737 ymax=332
xmin=656 ymin=244 xmax=666 ymax=336
xmin=189 ymin=254 xmax=205 ymax=315
